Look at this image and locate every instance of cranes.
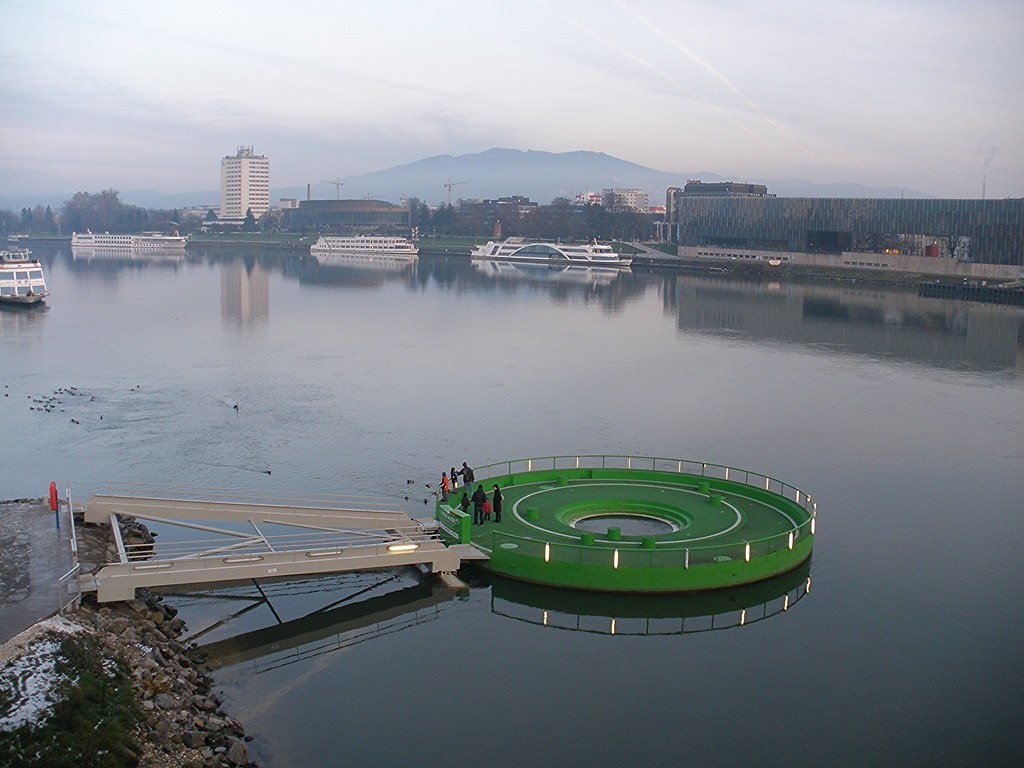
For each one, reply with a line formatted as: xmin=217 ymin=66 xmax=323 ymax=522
xmin=318 ymin=177 xmax=344 ymax=199
xmin=364 ymin=192 xmax=387 ymax=200
xmin=444 ymin=178 xmax=472 ymax=205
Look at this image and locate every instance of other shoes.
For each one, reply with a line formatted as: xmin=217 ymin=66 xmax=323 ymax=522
xmin=492 ymin=520 xmax=501 ymax=523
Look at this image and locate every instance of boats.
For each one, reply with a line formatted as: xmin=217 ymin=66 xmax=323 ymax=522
xmin=70 ymin=230 xmax=190 ymax=247
xmin=310 ymin=234 xmax=420 ymax=255
xmin=7 ymin=234 xmax=21 ymax=243
xmin=311 ymin=252 xmax=419 ymax=270
xmin=471 ymin=237 xmax=634 ymax=266
xmin=471 ymin=260 xmax=631 ymax=287
xmin=70 ymin=246 xmax=187 ymax=263
xmin=0 ymin=244 xmax=50 ymax=307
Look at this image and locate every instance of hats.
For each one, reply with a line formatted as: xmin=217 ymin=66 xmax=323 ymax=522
xmin=478 ymin=484 xmax=482 ymax=488
xmin=492 ymin=484 xmax=498 ymax=488
xmin=463 ymin=462 xmax=466 ymax=466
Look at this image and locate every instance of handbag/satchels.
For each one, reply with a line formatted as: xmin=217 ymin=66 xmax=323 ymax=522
xmin=500 ymin=493 xmax=504 ymax=501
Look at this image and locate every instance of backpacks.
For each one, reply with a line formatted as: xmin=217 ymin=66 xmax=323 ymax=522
xmin=476 ymin=494 xmax=484 ymax=507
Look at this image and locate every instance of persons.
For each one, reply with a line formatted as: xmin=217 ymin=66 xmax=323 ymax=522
xmin=451 ymin=462 xmax=502 ymax=526
xmin=438 ymin=471 xmax=449 ymax=502
